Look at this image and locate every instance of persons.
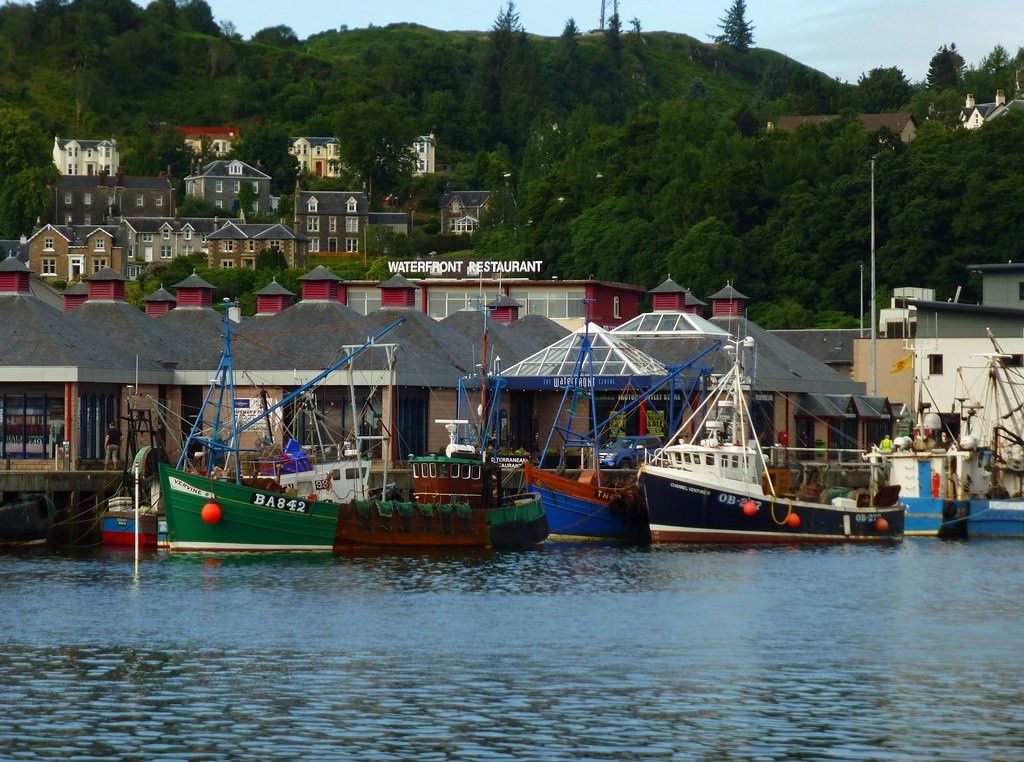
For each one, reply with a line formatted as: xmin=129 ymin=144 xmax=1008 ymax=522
xmin=879 ymin=434 xmax=894 ymax=452
xmin=103 ymin=422 xmax=125 ymax=470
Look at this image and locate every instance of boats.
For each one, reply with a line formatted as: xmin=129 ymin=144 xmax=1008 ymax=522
xmin=155 ymin=292 xmax=553 ymax=557
xmin=856 ymin=328 xmax=1024 ymax=545
xmin=525 ymin=299 xmax=724 ymax=547
xmin=95 ymin=397 xmax=158 ymax=547
xmin=629 ymin=281 xmax=908 ymax=551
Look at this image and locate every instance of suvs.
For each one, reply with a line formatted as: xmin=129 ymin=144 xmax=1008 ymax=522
xmin=597 ymin=436 xmax=663 ymax=469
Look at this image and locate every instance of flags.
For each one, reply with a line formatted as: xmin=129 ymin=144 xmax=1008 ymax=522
xmin=890 ymin=354 xmax=913 ymax=373
